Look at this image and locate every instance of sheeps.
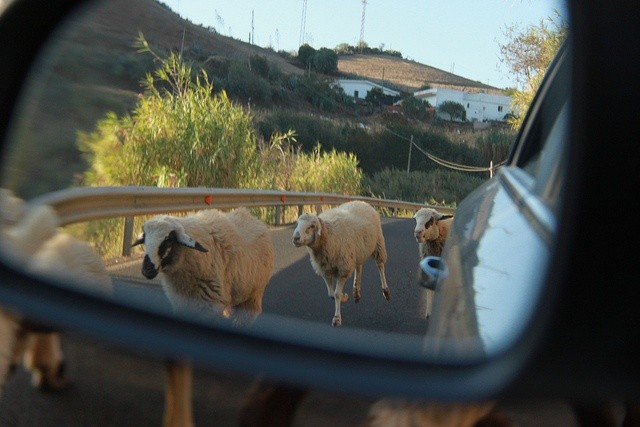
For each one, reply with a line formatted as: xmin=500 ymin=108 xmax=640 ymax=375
xmin=131 ymin=206 xmax=272 ymax=327
xmin=412 ymin=207 xmax=454 ymax=320
xmin=292 ymin=199 xmax=391 ymax=326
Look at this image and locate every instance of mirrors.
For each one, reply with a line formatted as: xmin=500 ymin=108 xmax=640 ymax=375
xmin=0 ymin=1 xmax=573 ymax=406
